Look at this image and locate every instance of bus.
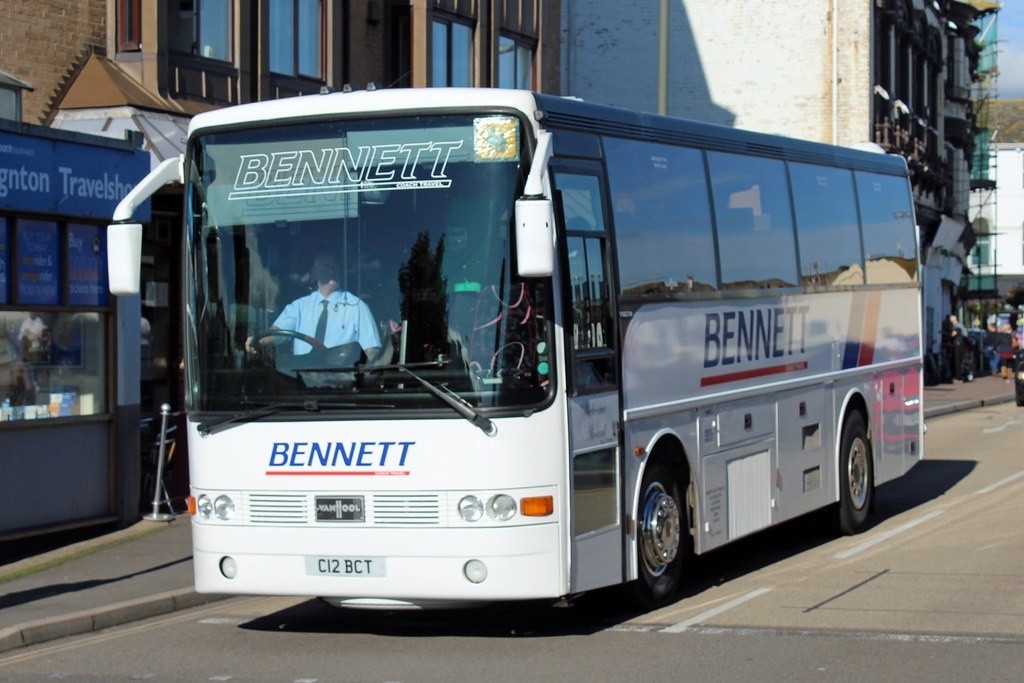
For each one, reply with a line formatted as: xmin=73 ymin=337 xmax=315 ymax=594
xmin=108 ymin=86 xmax=929 ymax=614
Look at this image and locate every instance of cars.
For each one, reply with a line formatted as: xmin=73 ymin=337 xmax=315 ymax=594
xmin=963 ymin=311 xmax=1016 ymax=372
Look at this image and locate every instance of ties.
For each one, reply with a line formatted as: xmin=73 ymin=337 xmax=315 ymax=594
xmin=311 ymin=299 xmax=329 ymax=352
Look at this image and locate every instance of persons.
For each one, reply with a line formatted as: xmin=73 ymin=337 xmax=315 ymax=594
xmin=949 ymin=314 xmax=970 ymax=383
xmin=16 ymin=312 xmax=48 ymax=353
xmin=972 ymin=314 xmax=982 ymax=329
xmin=245 ymin=251 xmax=382 ymax=377
xmin=984 ymin=313 xmax=1024 ymax=379
